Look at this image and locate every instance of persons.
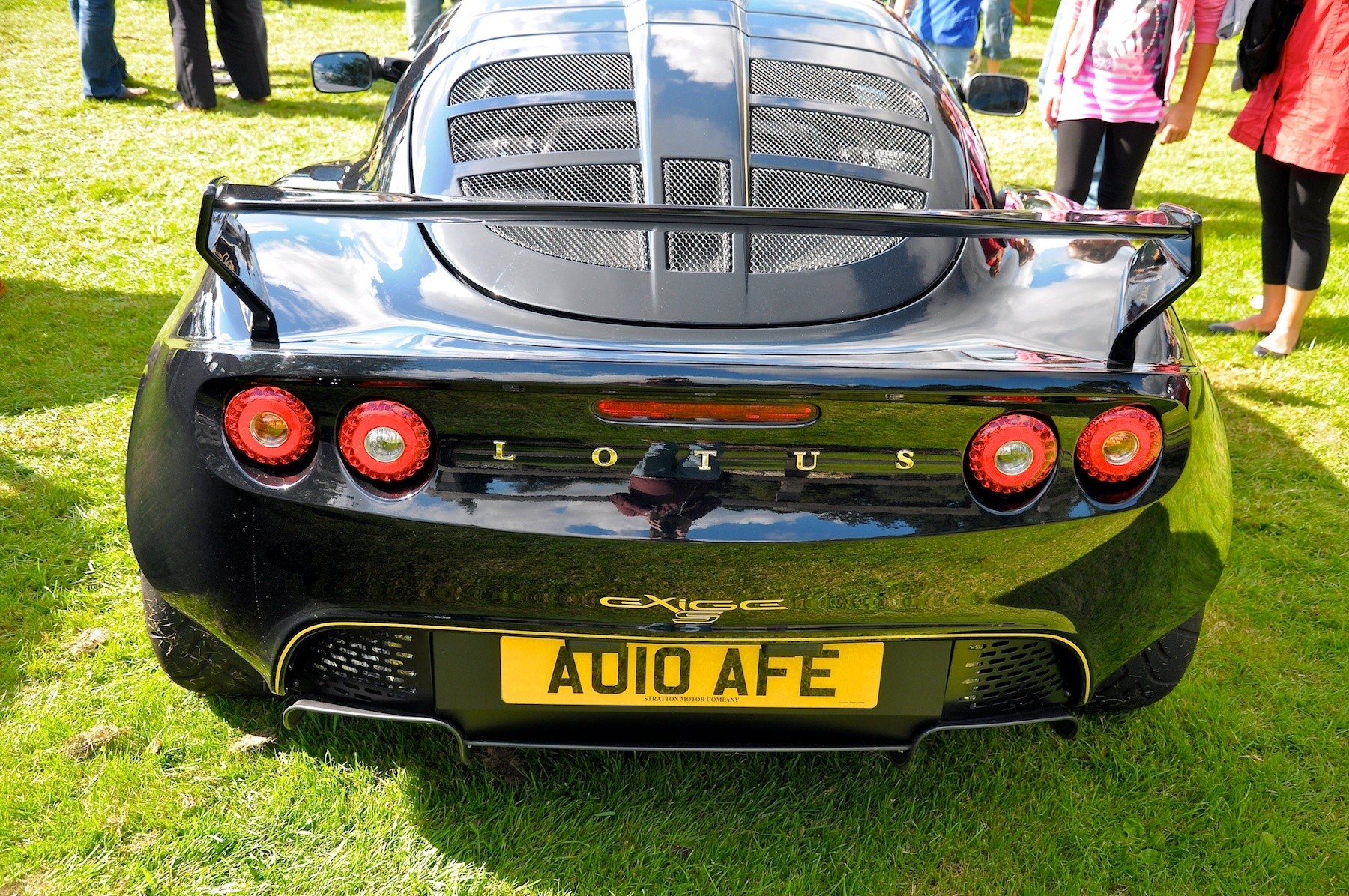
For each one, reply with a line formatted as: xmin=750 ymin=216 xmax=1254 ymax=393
xmin=908 ymin=0 xmax=983 ymax=79
xmin=611 ymin=437 xmax=725 ymax=540
xmin=68 ymin=0 xmax=149 ymax=101
xmin=1039 ymin=0 xmax=1227 ymax=211
xmin=406 ymin=0 xmax=443 ymax=60
xmin=965 ymin=0 xmax=1014 ymax=78
xmin=1206 ymin=0 xmax=1349 ymax=361
xmin=167 ymin=0 xmax=271 ymax=113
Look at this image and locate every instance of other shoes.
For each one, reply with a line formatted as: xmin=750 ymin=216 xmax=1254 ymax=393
xmin=121 ymin=86 xmax=148 ymax=99
xmin=1253 ymin=334 xmax=1301 ymax=360
xmin=211 ymin=60 xmax=227 ymax=71
xmin=214 ymin=73 xmax=233 ymax=84
xmin=1207 ymin=323 xmax=1270 ymax=335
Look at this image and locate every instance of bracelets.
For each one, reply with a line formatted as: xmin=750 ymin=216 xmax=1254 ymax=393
xmin=1048 ymin=73 xmax=1065 ymax=84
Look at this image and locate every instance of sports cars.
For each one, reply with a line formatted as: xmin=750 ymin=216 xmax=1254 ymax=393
xmin=121 ymin=0 xmax=1236 ymax=767
